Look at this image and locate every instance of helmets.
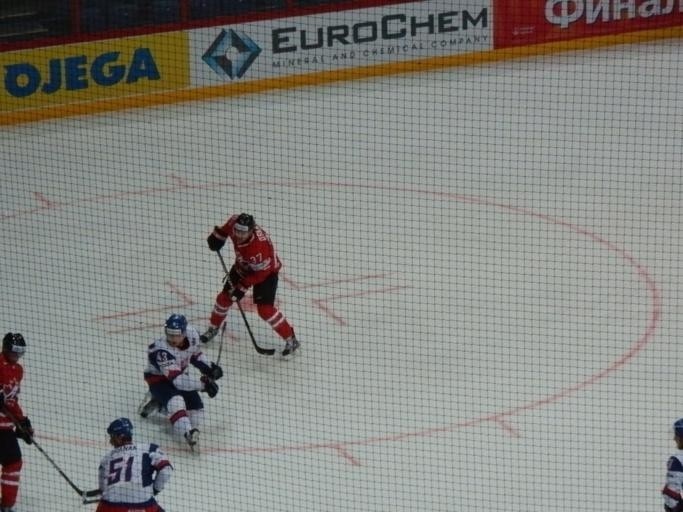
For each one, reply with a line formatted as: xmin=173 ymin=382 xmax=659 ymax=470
xmin=164 ymin=313 xmax=187 ymax=335
xmin=107 ymin=417 xmax=135 ymax=437
xmin=234 ymin=212 xmax=255 ymax=232
xmin=3 ymin=333 xmax=26 ymax=354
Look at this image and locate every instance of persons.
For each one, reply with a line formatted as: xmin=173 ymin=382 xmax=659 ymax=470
xmin=662 ymin=418 xmax=682 ymax=511
xmin=0 ymin=332 xmax=33 ymax=512
xmin=98 ymin=418 xmax=171 ymax=511
xmin=140 ymin=314 xmax=222 ymax=451
xmin=199 ymin=214 xmax=299 ymax=357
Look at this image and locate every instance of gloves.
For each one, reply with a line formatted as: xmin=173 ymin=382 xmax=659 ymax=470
xmin=14 ymin=416 xmax=34 ymax=444
xmin=200 ymin=361 xmax=222 ymax=398
xmin=229 ymin=279 xmax=247 ymax=302
xmin=207 ymin=225 xmax=227 ymax=252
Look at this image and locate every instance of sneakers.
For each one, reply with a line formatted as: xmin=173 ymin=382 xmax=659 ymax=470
xmin=184 ymin=428 xmax=199 ymax=453
xmin=140 ymin=395 xmax=162 ymax=418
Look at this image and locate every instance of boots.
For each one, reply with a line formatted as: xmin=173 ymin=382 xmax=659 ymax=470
xmin=281 ymin=328 xmax=301 ymax=356
xmin=200 ymin=325 xmax=219 ymax=342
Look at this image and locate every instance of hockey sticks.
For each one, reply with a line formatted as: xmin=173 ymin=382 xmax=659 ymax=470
xmin=216 ymin=250 xmax=275 ymax=355
xmin=1 ymin=404 xmax=101 ymax=497
xmin=82 ymin=489 xmax=101 ymax=504
